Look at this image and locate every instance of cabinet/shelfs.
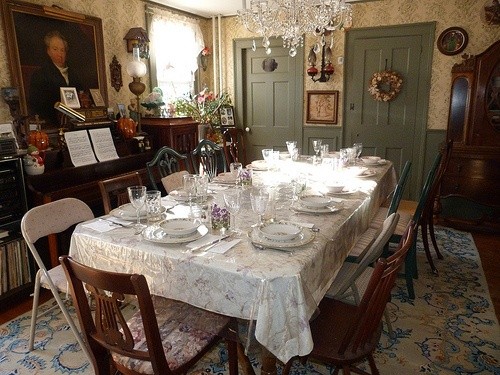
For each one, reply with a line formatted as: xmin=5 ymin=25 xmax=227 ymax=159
xmin=202 ymin=142 xmax=233 ymax=174
xmin=432 ymin=39 xmax=500 ymax=236
xmin=140 ymin=115 xmax=200 ymax=174
xmin=0 ymin=135 xmax=164 ymax=303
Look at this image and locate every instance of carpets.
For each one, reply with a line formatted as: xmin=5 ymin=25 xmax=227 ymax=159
xmin=0 ymin=224 xmax=500 ymax=375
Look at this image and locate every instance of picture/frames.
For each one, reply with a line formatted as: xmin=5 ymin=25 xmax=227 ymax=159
xmin=0 ymin=0 xmax=109 ymax=124
xmin=218 ymin=105 xmax=235 ymax=127
xmin=305 ymin=89 xmax=338 ymax=124
xmin=437 ymin=27 xmax=469 ymax=56
xmin=116 ymin=102 xmax=127 ymax=118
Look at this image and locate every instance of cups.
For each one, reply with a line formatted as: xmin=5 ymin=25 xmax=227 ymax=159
xmin=210 ymin=204 xmax=231 ymax=231
xmin=240 ymin=169 xmax=253 ymax=187
xmin=145 ymin=191 xmax=162 ymax=225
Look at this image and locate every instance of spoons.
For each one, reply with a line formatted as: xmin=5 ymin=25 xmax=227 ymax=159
xmin=251 ymin=242 xmax=295 ymax=254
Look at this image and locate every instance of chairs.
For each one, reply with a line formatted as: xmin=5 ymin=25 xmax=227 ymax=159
xmin=99 ymin=128 xmax=247 ymax=215
xmin=58 ymin=255 xmax=240 ymax=375
xmin=20 ymin=198 xmax=95 ymax=367
xmin=277 ymin=141 xmax=454 ymax=375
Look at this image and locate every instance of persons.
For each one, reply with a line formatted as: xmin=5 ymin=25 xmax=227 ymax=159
xmin=28 ymin=30 xmax=82 ymax=130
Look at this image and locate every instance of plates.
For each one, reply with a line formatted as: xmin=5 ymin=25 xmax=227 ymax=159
xmin=328 ymin=160 xmax=387 ymax=194
xmin=169 ymin=188 xmax=212 ymax=202
xmin=291 ymin=202 xmax=345 ymax=213
xmin=115 ymin=204 xmax=167 ymax=220
xmin=142 ymin=221 xmax=208 ymax=243
xmin=246 ymin=159 xmax=273 ymax=171
xmin=247 ymin=223 xmax=316 ymax=248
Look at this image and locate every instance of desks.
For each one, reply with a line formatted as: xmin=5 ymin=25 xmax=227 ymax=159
xmin=64 ymin=153 xmax=401 ymax=375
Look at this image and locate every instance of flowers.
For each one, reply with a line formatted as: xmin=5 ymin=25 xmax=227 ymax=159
xmin=368 ymin=71 xmax=403 ymax=103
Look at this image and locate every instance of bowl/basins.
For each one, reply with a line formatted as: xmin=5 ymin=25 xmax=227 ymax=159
xmin=299 ymin=195 xmax=333 ymax=209
xmin=258 ymin=222 xmax=302 ymax=240
xmin=159 ymin=218 xmax=201 ymax=235
xmin=362 ymin=156 xmax=381 ymax=163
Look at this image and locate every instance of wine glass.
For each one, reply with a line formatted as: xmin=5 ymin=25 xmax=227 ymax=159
xmin=261 ymin=138 xmax=362 ymax=197
xmin=183 ymin=173 xmax=208 ymax=219
xmin=249 ymin=190 xmax=269 ymax=228
xmin=223 ymin=189 xmax=241 ymax=237
xmin=230 ymin=164 xmax=242 ymax=188
xmin=127 ymin=185 xmax=146 ymax=229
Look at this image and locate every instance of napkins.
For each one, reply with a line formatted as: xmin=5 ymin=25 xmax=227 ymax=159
xmin=186 ymin=234 xmax=242 ymax=254
xmin=81 ymin=217 xmax=134 ymax=232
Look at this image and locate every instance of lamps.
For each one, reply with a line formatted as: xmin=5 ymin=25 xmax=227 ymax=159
xmin=236 ymin=0 xmax=353 ymax=58
xmin=306 ymin=42 xmax=335 ymax=82
xmin=1 ymin=87 xmax=26 ymax=136
xmin=126 ymin=47 xmax=149 ymax=136
